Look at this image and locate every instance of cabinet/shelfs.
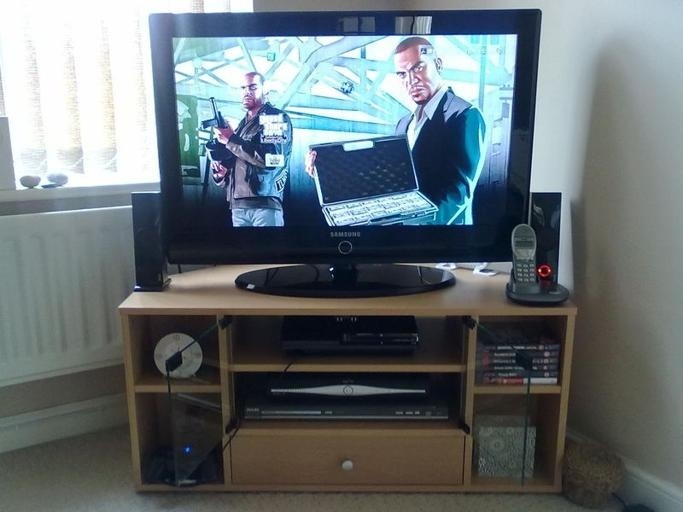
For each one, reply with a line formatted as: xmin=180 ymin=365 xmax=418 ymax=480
xmin=119 ymin=264 xmax=579 ymax=496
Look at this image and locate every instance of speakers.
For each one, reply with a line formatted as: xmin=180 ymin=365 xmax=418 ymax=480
xmin=528 ymin=191 xmax=562 ymax=294
xmin=131 ymin=192 xmax=171 ymax=291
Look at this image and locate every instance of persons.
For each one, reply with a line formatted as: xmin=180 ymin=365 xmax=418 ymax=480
xmin=208 ymin=71 xmax=295 ymax=225
xmin=304 ymin=34 xmax=491 ymax=225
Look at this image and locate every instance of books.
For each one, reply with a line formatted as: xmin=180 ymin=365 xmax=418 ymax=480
xmin=475 ymin=325 xmax=561 ymax=385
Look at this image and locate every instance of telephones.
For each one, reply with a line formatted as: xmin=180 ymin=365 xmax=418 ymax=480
xmin=506 ymin=224 xmax=569 ymax=304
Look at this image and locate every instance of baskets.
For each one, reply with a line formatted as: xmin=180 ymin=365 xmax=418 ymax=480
xmin=562 ymin=437 xmax=626 ymax=508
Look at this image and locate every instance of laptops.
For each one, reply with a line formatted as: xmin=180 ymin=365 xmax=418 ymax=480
xmin=309 ymin=133 xmax=439 ymax=227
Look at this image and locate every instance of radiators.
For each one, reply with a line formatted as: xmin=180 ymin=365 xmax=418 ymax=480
xmin=0 ymin=206 xmax=134 ymax=389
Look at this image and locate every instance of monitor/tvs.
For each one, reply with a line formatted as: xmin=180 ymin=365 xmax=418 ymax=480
xmin=148 ymin=8 xmax=542 ymax=299
